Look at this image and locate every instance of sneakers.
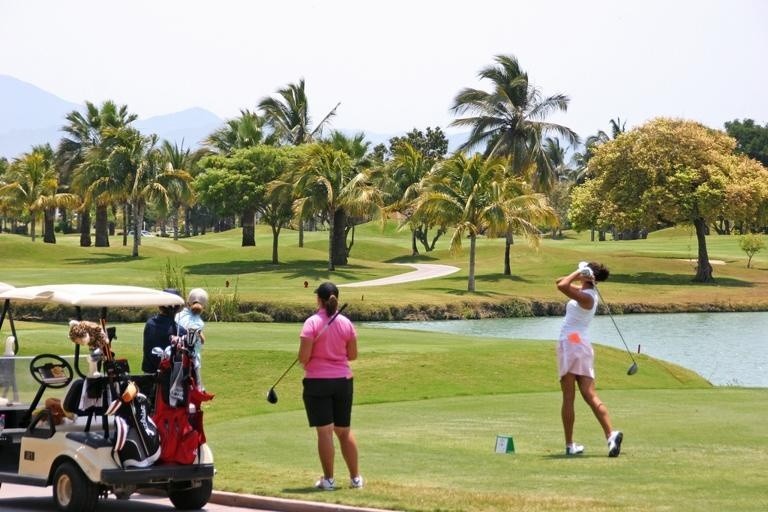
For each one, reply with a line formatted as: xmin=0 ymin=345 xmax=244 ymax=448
xmin=352 ymin=476 xmax=363 ymax=487
xmin=608 ymin=432 xmax=623 ymax=457
xmin=566 ymin=444 xmax=584 ymax=455
xmin=316 ymin=476 xmax=335 ymax=490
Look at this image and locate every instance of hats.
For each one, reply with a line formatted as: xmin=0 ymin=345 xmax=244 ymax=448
xmin=314 ymin=283 xmax=339 ymax=294
xmin=188 ymin=288 xmax=208 ymax=307
xmin=578 ymin=261 xmax=593 ymax=278
xmin=159 ymin=289 xmax=180 ymax=310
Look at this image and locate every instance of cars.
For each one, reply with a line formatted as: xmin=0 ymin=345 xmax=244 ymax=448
xmin=128 ymin=230 xmax=153 ymax=237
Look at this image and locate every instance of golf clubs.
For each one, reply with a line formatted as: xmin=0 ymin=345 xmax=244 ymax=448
xmin=99 ymin=314 xmax=115 ymax=361
xmin=169 ymin=325 xmax=202 ymax=355
xmin=266 ymin=302 xmax=348 ymax=404
xmin=590 ymin=278 xmax=638 ymax=376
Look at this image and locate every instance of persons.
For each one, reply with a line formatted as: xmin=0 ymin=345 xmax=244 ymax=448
xmin=555 ymin=262 xmax=623 ymax=457
xmin=142 ymin=287 xmax=209 ymax=407
xmin=298 ymin=282 xmax=363 ymax=490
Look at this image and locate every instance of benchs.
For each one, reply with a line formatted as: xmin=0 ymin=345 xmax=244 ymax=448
xmin=25 ymin=375 xmax=154 ymax=433
